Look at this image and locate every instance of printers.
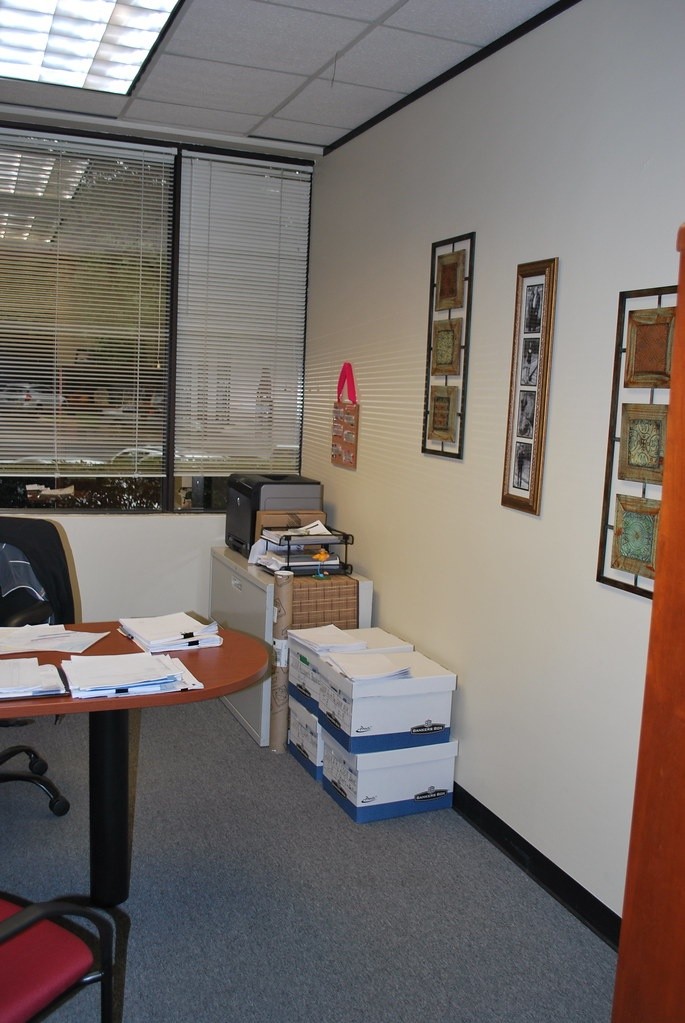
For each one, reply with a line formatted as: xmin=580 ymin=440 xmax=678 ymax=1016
xmin=225 ymin=474 xmax=324 ymax=560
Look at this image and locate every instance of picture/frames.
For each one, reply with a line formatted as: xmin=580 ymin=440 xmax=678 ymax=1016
xmin=431 ymin=317 xmax=463 ymax=376
xmin=611 ymin=494 xmax=661 ymax=581
xmin=499 ymin=256 xmax=557 ymax=516
xmin=428 ymin=384 xmax=460 ymax=443
xmin=618 ymin=402 xmax=667 ymax=485
xmin=624 ymin=307 xmax=675 ymax=388
xmin=435 ymin=249 xmax=465 ymax=310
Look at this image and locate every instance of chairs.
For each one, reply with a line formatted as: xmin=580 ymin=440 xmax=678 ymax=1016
xmin=0 ymin=516 xmax=82 ymax=816
xmin=0 ymin=882 xmax=130 ymax=1023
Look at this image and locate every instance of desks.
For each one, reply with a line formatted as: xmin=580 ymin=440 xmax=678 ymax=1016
xmin=0 ymin=612 xmax=276 ymax=904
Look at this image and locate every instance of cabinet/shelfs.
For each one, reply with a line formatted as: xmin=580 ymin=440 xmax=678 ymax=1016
xmin=209 ymin=546 xmax=373 ymax=751
xmin=256 ymin=526 xmax=353 ymax=578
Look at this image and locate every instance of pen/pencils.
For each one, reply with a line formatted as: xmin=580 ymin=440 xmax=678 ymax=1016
xmin=118 ymin=625 xmax=134 ymax=641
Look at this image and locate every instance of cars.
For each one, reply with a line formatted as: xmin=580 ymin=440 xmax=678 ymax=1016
xmin=0 ymin=383 xmax=68 ymax=414
xmin=101 ymin=405 xmax=207 ymax=443
xmin=112 ymin=446 xmax=182 ymax=464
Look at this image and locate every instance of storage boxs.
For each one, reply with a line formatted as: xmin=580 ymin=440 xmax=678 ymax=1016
xmin=321 ymin=729 xmax=458 ymax=824
xmin=317 ymin=652 xmax=457 ymax=753
xmin=287 ymin=628 xmax=415 ymax=713
xmin=284 ymin=685 xmax=324 ymax=782
